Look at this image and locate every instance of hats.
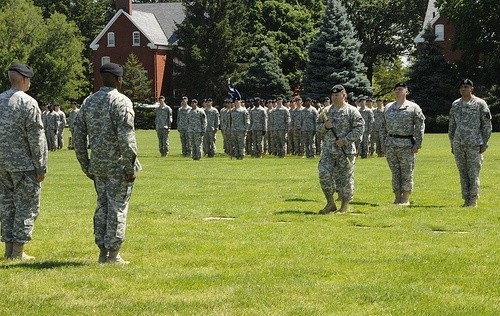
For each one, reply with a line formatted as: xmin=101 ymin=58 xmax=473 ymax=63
xmin=347 ymin=92 xmax=396 ymax=104
xmin=159 ymin=96 xmax=212 ymax=102
xmin=9 ymin=63 xmax=35 ymax=78
xmin=223 ymin=95 xmax=329 ymax=104
xmin=99 ymin=62 xmax=124 ymax=77
xmin=330 ymin=84 xmax=344 ymax=94
xmin=458 ymin=78 xmax=473 ymax=90
xmin=39 ymin=99 xmax=77 ymax=108
xmin=394 ymin=82 xmax=407 ymax=91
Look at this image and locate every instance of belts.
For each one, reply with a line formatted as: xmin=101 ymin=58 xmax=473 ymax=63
xmin=389 ymin=134 xmax=413 ymax=138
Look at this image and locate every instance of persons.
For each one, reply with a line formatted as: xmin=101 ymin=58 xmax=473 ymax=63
xmin=383 ymin=82 xmax=426 ymax=205
xmin=448 ymin=79 xmax=492 ymax=207
xmin=186 ymin=99 xmax=208 ymax=160
xmin=177 ymin=97 xmax=192 ymax=157
xmin=42 ymin=103 xmax=66 ymax=151
xmin=67 ymin=101 xmax=80 ymax=150
xmin=0 ymin=63 xmax=48 ymax=260
xmin=315 ymin=85 xmax=366 ymax=214
xmin=73 ymin=63 xmax=142 ymax=264
xmin=220 ymin=93 xmax=388 ymax=160
xmin=155 ymin=96 xmax=173 ymax=156
xmin=203 ymin=98 xmax=220 ymax=157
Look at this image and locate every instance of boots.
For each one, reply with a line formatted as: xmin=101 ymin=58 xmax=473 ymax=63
xmin=462 ymin=195 xmax=478 ymax=208
xmin=391 ymin=192 xmax=411 ymax=206
xmin=4 ymin=243 xmax=36 ymax=260
xmin=98 ymin=246 xmax=130 ymax=265
xmin=319 ymin=195 xmax=337 ymax=214
xmin=334 ymin=197 xmax=351 ymax=214
